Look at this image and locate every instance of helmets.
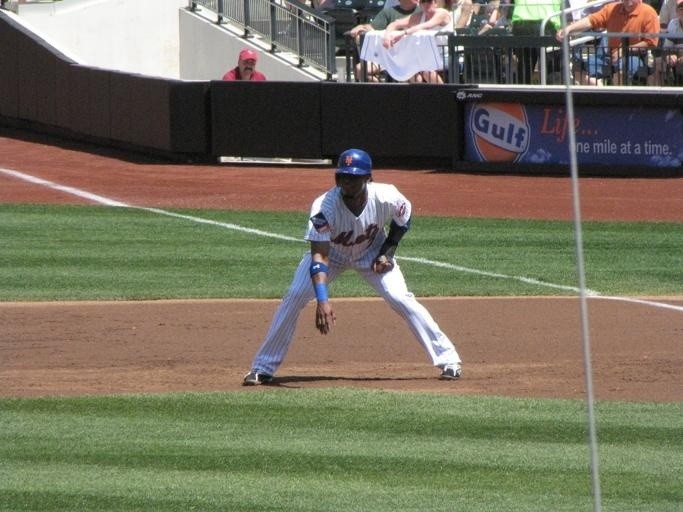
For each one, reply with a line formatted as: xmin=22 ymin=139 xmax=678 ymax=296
xmin=335 ymin=149 xmax=373 ymax=176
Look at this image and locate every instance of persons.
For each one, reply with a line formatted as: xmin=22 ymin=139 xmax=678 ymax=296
xmin=242 ymin=147 xmax=464 ymax=385
xmin=282 ymin=1 xmax=682 ymax=86
xmin=221 ymin=47 xmax=266 ymax=82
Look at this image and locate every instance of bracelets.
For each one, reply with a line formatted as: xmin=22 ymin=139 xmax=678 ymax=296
xmin=311 ymin=283 xmax=329 ymax=302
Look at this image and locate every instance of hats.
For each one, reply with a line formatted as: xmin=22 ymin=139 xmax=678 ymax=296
xmin=238 ymin=48 xmax=257 ymax=60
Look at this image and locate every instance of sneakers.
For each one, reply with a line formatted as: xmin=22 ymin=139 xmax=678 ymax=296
xmin=439 ymin=364 xmax=463 ymax=381
xmin=243 ymin=369 xmax=275 ymax=386
